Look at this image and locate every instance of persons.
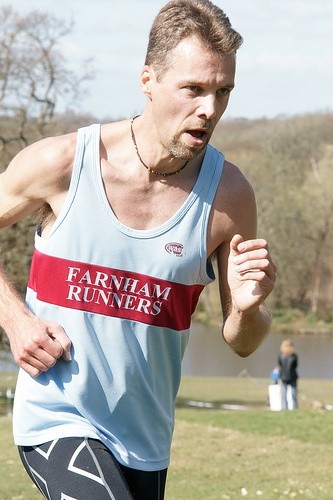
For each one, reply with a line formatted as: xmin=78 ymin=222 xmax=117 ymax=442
xmin=0 ymin=0 xmax=276 ymax=500
xmin=276 ymin=340 xmax=301 ymax=410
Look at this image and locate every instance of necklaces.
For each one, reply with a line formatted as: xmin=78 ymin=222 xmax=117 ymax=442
xmin=130 ymin=116 xmax=191 ymax=177
